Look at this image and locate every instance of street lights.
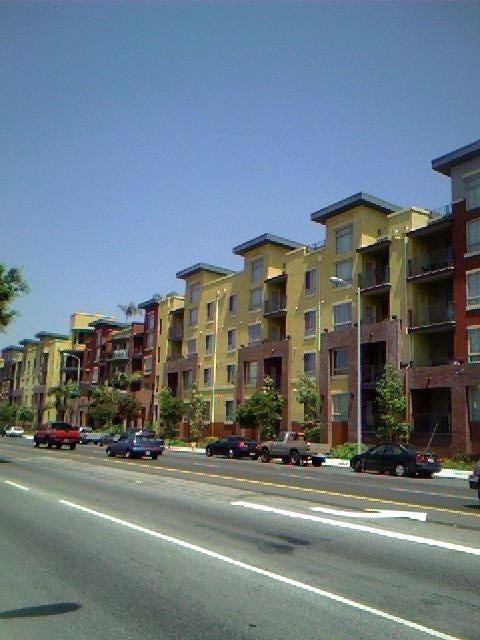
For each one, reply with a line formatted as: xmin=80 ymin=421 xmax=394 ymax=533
xmin=330 ymin=277 xmax=361 ymax=455
xmin=63 ymin=352 xmax=80 ymax=427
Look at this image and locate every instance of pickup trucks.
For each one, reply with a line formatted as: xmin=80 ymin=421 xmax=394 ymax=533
xmin=259 ymin=430 xmax=336 ymax=467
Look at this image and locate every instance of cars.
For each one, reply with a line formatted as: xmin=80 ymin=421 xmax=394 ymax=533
xmin=203 ymin=431 xmax=257 ymax=459
xmin=5 ymin=422 xmax=164 ymax=460
xmin=350 ymin=442 xmax=442 ymax=477
